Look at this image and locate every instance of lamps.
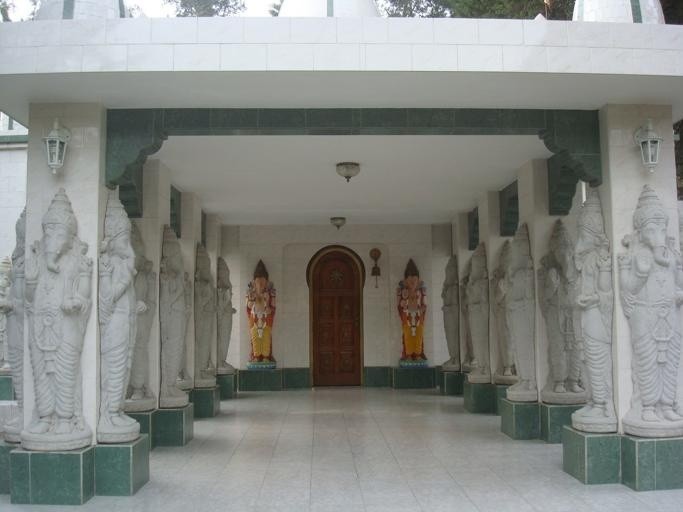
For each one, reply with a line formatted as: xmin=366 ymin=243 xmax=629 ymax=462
xmin=336 ymin=162 xmax=360 ymax=183
xmin=330 ymin=217 xmax=346 ymax=230
xmin=633 ymin=118 xmax=664 ymax=173
xmin=41 ymin=117 xmax=69 ymax=175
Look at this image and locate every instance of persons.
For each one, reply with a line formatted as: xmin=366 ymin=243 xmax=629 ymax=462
xmin=4 ymin=185 xmax=159 ymax=436
xmin=441 ymin=255 xmax=460 ymax=371
xmin=397 ymin=258 xmax=428 ymax=361
xmin=196 ymin=244 xmax=216 ymax=387
xmin=160 ymin=224 xmax=189 ymax=406
xmin=217 ymin=257 xmax=237 ymax=375
xmin=245 ymin=259 xmax=277 ymax=363
